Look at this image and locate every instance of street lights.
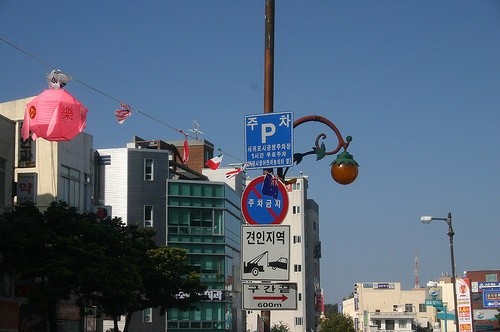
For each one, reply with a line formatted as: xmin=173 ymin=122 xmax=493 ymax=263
xmin=420 ymin=212 xmax=460 ymax=332
xmin=260 ymin=113 xmax=360 ymax=332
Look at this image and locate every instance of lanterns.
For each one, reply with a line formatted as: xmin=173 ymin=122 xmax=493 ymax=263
xmin=21 ymin=70 xmax=87 ymax=142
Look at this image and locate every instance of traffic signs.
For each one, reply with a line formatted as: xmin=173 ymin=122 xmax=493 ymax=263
xmin=241 ymin=282 xmax=298 ymax=311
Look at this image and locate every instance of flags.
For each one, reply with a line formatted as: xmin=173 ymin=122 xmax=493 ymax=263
xmin=115 ymin=102 xmax=132 ymax=125
xmin=226 ymin=162 xmax=252 ymax=179
xmin=178 ymin=129 xmax=189 ymax=164
xmin=261 ymin=172 xmax=278 ymax=199
xmin=205 ymin=148 xmax=224 ymax=170
